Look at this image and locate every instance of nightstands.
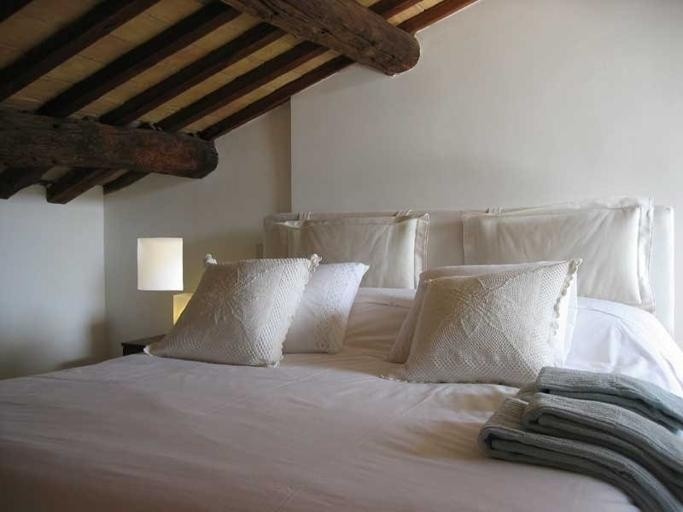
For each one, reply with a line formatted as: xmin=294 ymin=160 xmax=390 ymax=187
xmin=121 ymin=334 xmax=164 ymax=355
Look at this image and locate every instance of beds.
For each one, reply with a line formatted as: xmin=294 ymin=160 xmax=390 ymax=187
xmin=0 ymin=201 xmax=683 ymax=512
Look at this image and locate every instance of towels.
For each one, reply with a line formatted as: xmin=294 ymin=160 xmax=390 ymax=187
xmin=476 ymin=366 xmax=683 ymax=512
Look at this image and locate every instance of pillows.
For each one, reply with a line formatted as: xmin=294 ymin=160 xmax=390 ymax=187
xmin=149 ymin=253 xmax=321 ymax=371
xmin=263 ymin=210 xmax=433 ymax=291
xmin=388 ymin=257 xmax=582 ymax=386
xmin=286 ymin=260 xmax=370 ymax=355
xmin=385 ymin=262 xmax=551 ymax=367
xmin=459 ymin=200 xmax=656 ymax=310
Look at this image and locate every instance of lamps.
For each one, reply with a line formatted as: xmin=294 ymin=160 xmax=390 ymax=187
xmin=136 ymin=236 xmax=193 ymax=326
